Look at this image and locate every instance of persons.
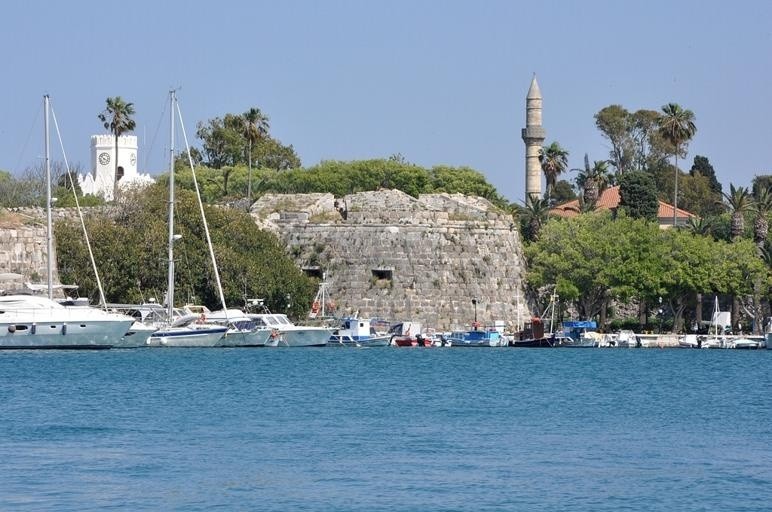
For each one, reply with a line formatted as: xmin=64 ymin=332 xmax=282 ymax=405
xmin=311 ymin=301 xmax=354 ymax=318
xmin=687 ymin=319 xmax=707 ymax=334
xmin=734 ymin=319 xmax=743 ymax=334
xmin=596 ymin=319 xmax=617 ymax=334
xmin=272 ymin=327 xmax=279 ymax=340
xmin=441 ymin=332 xmax=447 ymax=346
